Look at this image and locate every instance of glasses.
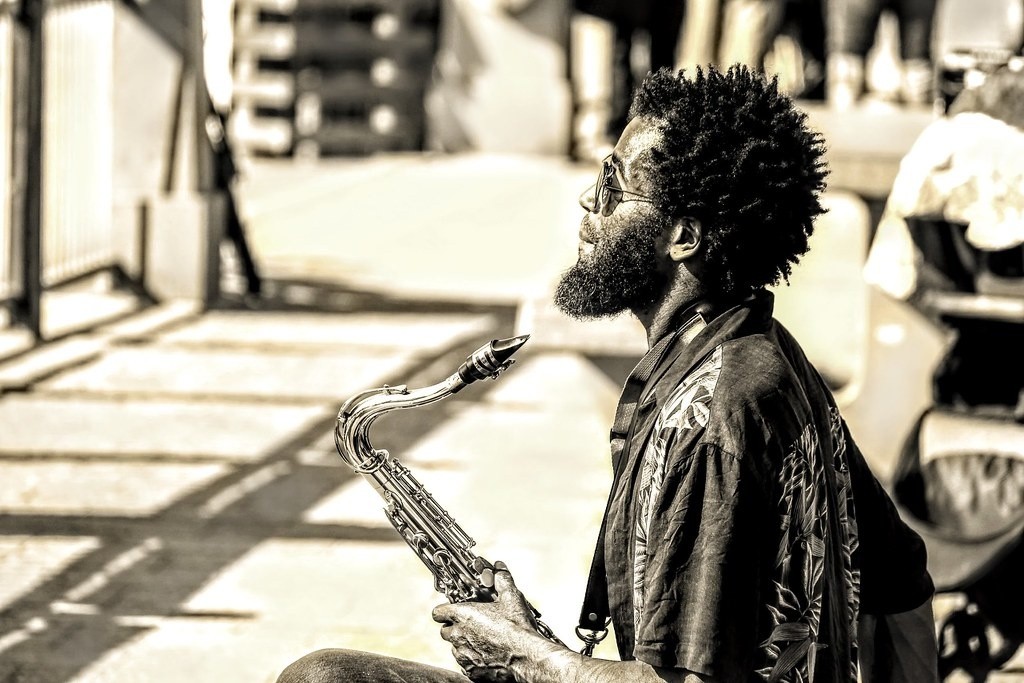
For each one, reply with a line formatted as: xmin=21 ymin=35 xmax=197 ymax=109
xmin=595 ymin=153 xmax=653 ymax=208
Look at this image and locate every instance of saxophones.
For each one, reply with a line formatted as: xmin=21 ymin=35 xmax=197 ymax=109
xmin=333 ymin=333 xmax=568 ymax=683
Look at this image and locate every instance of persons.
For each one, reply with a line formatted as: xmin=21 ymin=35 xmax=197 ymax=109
xmin=276 ymin=62 xmax=938 ymax=683
